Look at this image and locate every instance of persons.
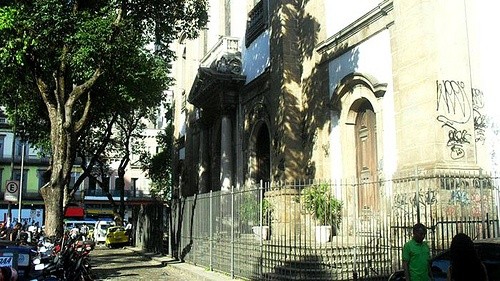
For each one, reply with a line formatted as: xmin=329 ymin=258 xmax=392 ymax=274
xmin=62 ymin=223 xmax=89 ymax=244
xmin=0 ymin=218 xmax=44 ymax=242
xmin=125 ymin=217 xmax=135 ymax=246
xmin=447 ymin=232 xmax=489 ymax=281
xmin=402 ymin=223 xmax=434 ymax=281
xmin=116 ymin=213 xmax=122 ymax=226
xmin=93 ymin=218 xmax=101 ymax=247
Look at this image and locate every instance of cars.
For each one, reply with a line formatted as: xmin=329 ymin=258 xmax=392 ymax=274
xmin=105 ymin=226 xmax=130 ymax=248
xmin=387 ymin=235 xmax=500 ymax=281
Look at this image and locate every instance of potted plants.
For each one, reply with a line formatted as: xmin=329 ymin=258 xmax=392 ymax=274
xmin=240 ymin=192 xmax=275 ymax=240
xmin=300 ymin=181 xmax=345 ymax=244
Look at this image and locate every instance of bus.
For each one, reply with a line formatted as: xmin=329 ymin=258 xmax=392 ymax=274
xmin=65 ymin=220 xmax=96 ymax=238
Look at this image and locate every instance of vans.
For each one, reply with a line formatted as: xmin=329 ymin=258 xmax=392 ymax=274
xmin=94 ymin=220 xmax=116 ymax=244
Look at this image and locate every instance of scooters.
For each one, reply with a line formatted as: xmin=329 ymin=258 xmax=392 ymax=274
xmin=0 ymin=230 xmax=99 ymax=281
